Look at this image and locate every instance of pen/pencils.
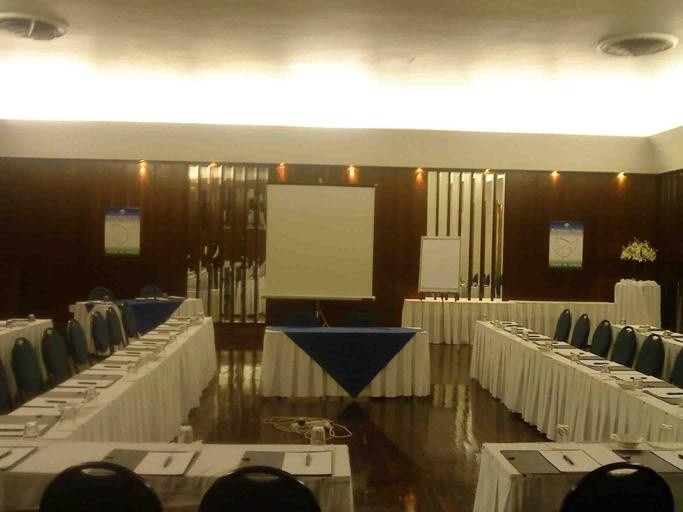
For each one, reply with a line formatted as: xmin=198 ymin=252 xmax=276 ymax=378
xmin=44 ymin=400 xmax=69 ymax=403
xmin=78 ymin=382 xmax=97 ymax=384
xmin=629 ymin=377 xmax=648 ymax=380
xmin=0 ymin=449 xmax=12 ymax=460
xmin=104 ymin=365 xmax=121 ymax=368
xmin=593 ymin=362 xmax=611 ymax=365
xmin=305 ymin=454 xmax=310 ymax=466
xmin=563 ymin=454 xmax=576 ymax=467
xmin=158 ymin=331 xmax=170 ymax=334
xmin=126 ymin=351 xmax=142 ymax=355
xmin=163 ymin=456 xmax=173 ymax=468
xmin=665 ymin=392 xmax=682 ymax=395
xmin=527 ymin=335 xmax=541 ymax=338
xmin=569 ymin=353 xmax=586 ymax=354
xmin=143 ymin=343 xmax=158 ymax=344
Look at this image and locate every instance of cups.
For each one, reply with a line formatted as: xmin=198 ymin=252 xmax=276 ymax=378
xmin=310 ymin=425 xmax=325 ymax=448
xmin=481 ymin=313 xmax=683 ymax=448
xmin=22 ymin=312 xmax=204 ymax=450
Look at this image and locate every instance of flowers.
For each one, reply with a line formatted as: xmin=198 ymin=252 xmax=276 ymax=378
xmin=619 ymin=238 xmax=659 ymax=281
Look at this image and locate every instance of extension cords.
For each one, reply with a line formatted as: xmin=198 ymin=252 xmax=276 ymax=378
xmin=289 ymin=421 xmax=330 ymax=431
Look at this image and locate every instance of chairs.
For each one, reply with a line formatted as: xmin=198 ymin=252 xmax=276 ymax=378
xmin=281 ymin=311 xmax=320 ymax=327
xmin=139 ymin=282 xmax=163 ymax=297
xmin=38 ymin=461 xmax=166 ymax=512
xmin=558 ymin=462 xmax=673 ymax=511
xmin=0 ymin=302 xmax=141 ymax=413
xmin=88 ymin=286 xmax=114 ymax=301
xmin=198 ymin=466 xmax=323 ymax=511
xmin=554 ymin=309 xmax=682 ymax=389
xmin=346 ymin=311 xmax=386 ymax=328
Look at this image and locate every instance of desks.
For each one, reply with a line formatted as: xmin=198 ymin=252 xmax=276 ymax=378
xmin=257 ymin=326 xmax=432 ymax=401
xmin=401 ymin=279 xmax=661 ymax=347
xmin=606 ymin=323 xmax=682 ymax=382
xmin=0 ymin=315 xmax=219 ymax=444
xmin=469 ymin=319 xmax=683 ymax=443
xmin=613 ymin=278 xmax=661 ymax=331
xmin=472 ymin=441 xmax=682 ymax=512
xmin=70 ymin=296 xmax=205 ymax=356
xmin=1 ymin=443 xmax=356 ymax=512
